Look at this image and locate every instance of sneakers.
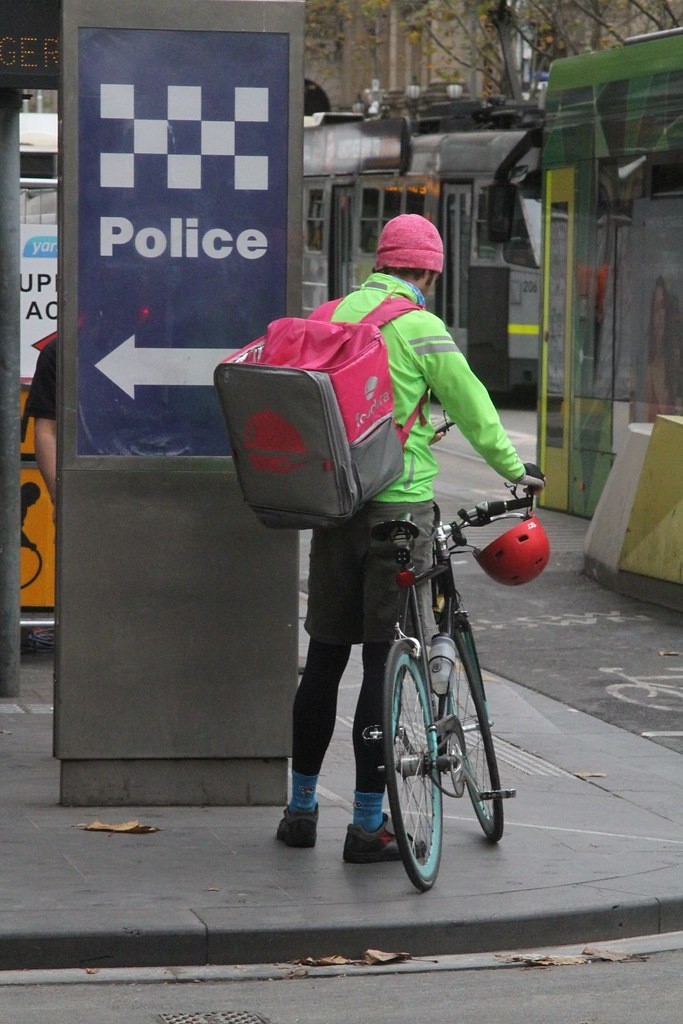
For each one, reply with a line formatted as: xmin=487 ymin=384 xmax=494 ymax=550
xmin=343 ymin=812 xmax=427 ymax=864
xmin=277 ymin=803 xmax=319 ymax=848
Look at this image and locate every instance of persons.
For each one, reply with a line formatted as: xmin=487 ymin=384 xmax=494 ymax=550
xmin=276 ymin=210 xmax=548 ymax=866
xmin=643 ymin=276 xmax=683 ymax=424
xmin=23 ymin=336 xmax=58 ymax=503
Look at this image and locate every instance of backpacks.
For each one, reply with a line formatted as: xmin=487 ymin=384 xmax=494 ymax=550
xmin=214 ymin=291 xmax=431 ymax=531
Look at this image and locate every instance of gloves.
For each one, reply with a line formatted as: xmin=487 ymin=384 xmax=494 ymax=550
xmin=517 ymin=463 xmax=546 ymax=496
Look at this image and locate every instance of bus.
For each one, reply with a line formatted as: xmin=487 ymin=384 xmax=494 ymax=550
xmin=19 ymin=84 xmax=615 ymax=402
xmin=474 ymin=30 xmax=683 ymax=516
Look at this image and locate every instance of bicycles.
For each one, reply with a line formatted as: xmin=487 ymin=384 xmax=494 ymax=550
xmin=364 ymin=478 xmax=533 ymax=892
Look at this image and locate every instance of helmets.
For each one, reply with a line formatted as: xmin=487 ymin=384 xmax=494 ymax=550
xmin=473 ymin=511 xmax=549 ymax=587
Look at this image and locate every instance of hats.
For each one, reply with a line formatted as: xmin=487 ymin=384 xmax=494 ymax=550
xmin=376 ymin=213 xmax=444 ymax=274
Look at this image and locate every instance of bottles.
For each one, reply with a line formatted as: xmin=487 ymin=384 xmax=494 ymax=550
xmin=428 ymin=632 xmax=456 ymax=695
